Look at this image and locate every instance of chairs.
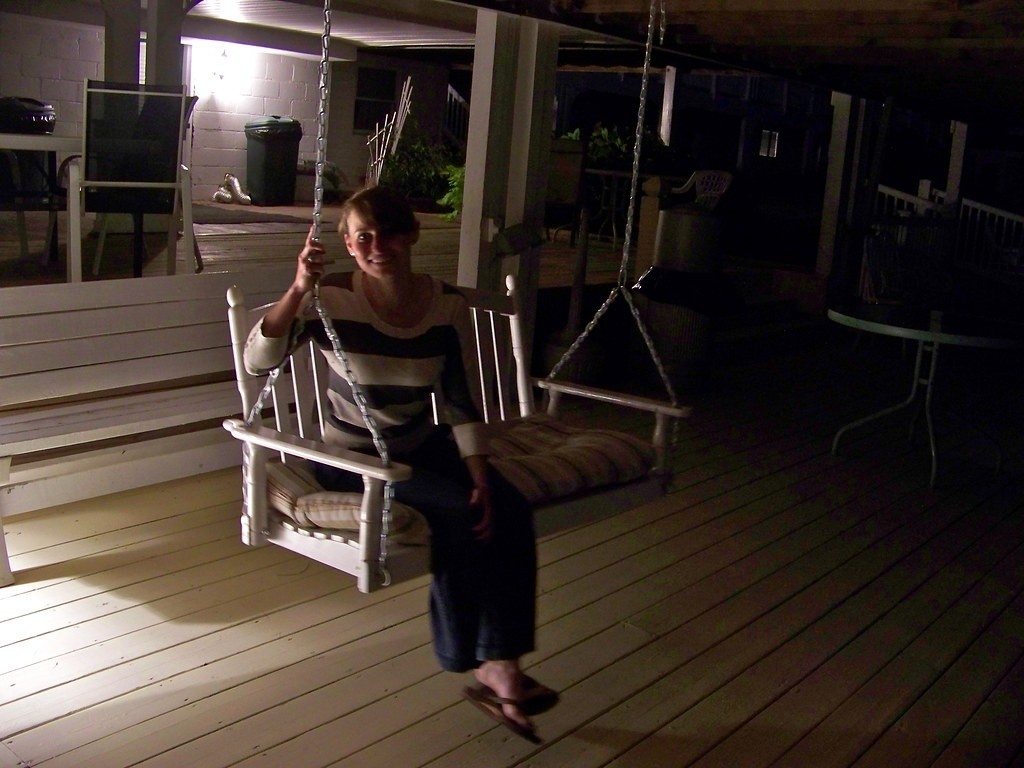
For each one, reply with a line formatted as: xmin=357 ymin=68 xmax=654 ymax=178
xmin=62 ymin=78 xmax=188 ymax=283
xmin=0 ymin=96 xmax=66 ymax=271
xmin=90 ymin=94 xmax=204 ymax=279
xmin=670 ymin=170 xmax=733 ymax=209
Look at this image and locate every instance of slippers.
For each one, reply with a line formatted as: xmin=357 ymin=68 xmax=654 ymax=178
xmin=460 ymin=684 xmax=541 ymax=745
xmin=520 ymin=673 xmax=560 ymax=716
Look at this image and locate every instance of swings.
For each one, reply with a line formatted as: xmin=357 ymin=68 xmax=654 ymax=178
xmin=221 ymin=1 xmax=697 ymax=596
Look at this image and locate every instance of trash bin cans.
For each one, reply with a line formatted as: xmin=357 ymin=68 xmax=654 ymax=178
xmin=1 ymin=95 xmax=57 ymax=208
xmin=245 ymin=120 xmax=303 ymax=206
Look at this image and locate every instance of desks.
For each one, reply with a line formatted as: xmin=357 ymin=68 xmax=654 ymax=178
xmin=826 ymin=305 xmax=1005 ymax=486
xmin=582 ymin=165 xmax=654 ymax=253
xmin=0 ymin=131 xmax=182 ymax=273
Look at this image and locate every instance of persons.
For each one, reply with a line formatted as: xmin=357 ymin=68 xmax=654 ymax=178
xmin=243 ymin=185 xmax=561 ymax=745
